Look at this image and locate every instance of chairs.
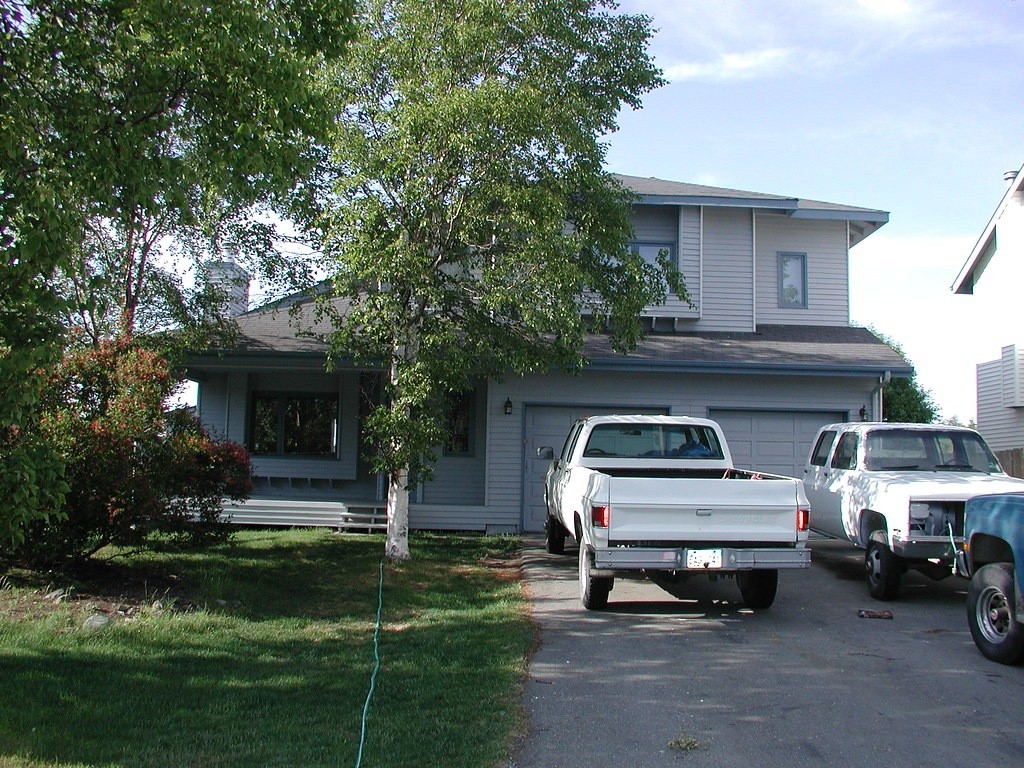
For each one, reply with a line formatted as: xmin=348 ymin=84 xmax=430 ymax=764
xmin=643 ymin=451 xmax=678 ymax=457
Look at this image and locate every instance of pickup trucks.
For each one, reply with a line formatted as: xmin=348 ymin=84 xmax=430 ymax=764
xmin=801 ymin=421 xmax=1024 ymax=600
xmin=536 ymin=415 xmax=811 ymax=611
xmin=952 ymin=491 xmax=1024 ymax=664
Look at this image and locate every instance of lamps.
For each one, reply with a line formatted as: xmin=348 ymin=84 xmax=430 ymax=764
xmin=860 ymin=405 xmax=868 ymax=422
xmin=504 ymin=397 xmax=512 ymax=414
